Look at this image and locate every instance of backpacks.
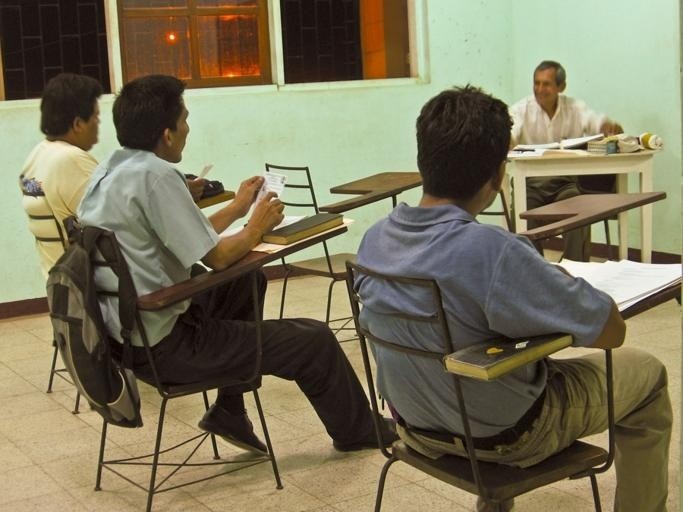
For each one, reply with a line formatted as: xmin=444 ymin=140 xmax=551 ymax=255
xmin=42 ymin=213 xmax=147 ymax=430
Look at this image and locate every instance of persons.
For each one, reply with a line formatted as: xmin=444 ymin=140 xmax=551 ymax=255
xmin=354 ymin=85 xmax=672 ymax=512
xmin=505 ymin=60 xmax=624 ymax=263
xmin=74 ymin=73 xmax=399 ymax=454
xmin=18 ymin=73 xmax=209 ymax=278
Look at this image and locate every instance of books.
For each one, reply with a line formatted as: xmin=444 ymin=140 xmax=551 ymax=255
xmin=262 ymin=211 xmax=342 ymax=246
xmin=512 ymin=133 xmax=607 ymax=153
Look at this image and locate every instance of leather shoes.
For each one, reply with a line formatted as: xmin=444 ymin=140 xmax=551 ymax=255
xmin=199 ymin=402 xmax=268 ymax=456
xmin=333 ymin=411 xmax=399 ymax=452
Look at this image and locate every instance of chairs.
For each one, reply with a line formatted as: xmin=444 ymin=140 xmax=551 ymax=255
xmin=20 ymin=190 xmax=81 ymax=416
xmin=59 ymin=217 xmax=283 ymax=512
xmin=344 ymin=263 xmax=615 ymax=512
xmin=262 ymin=166 xmax=358 ymax=342
xmin=475 ymin=188 xmax=569 ymax=265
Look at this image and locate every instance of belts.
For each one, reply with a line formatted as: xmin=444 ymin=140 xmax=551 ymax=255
xmin=399 ymin=388 xmax=546 ymax=449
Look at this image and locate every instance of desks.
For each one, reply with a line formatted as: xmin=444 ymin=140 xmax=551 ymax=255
xmin=509 ymin=146 xmax=663 ymax=268
